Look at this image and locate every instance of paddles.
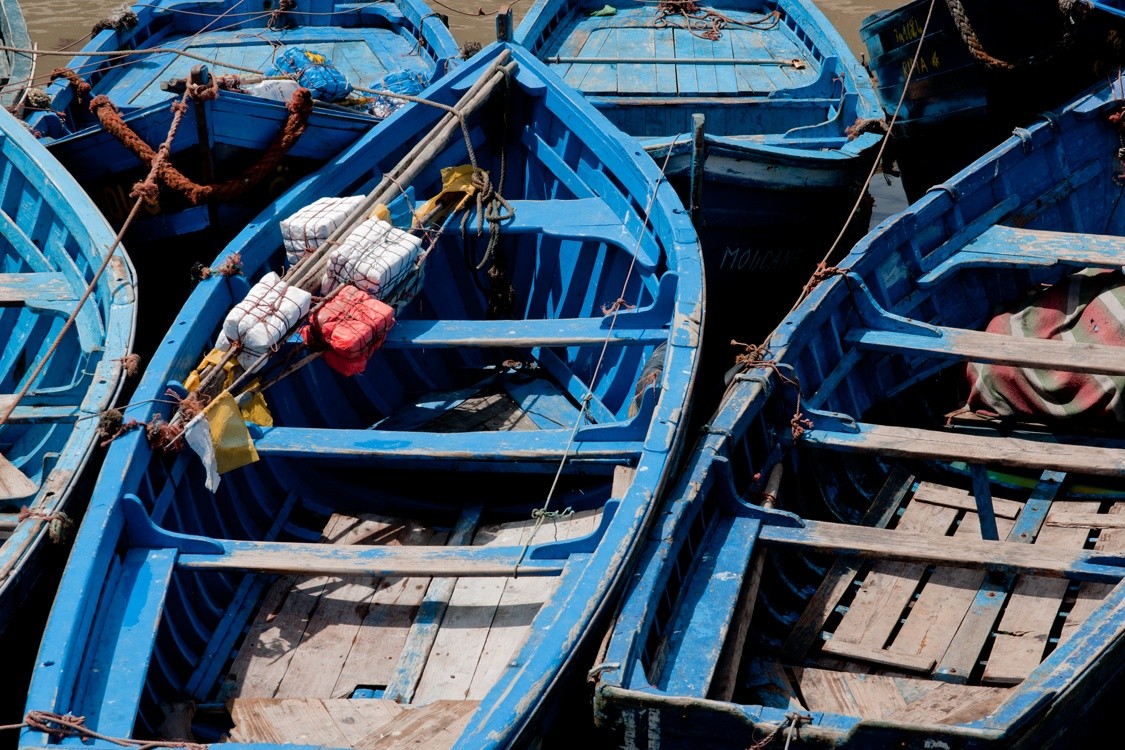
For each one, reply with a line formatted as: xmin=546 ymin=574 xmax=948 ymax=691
xmin=0 ymin=451 xmax=40 ymax=500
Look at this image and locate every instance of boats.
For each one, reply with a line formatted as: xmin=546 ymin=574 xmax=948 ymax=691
xmin=0 ymin=100 xmax=137 ymax=592
xmin=854 ymin=0 xmax=1124 ymax=123
xmin=508 ymin=0 xmax=894 ymax=230
xmin=593 ymin=75 xmax=1124 ymax=748
xmin=23 ymin=0 xmax=708 ymax=748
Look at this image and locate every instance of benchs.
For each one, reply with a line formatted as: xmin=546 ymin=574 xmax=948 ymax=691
xmin=0 ymin=240 xmax=105 ymax=423
xmin=843 ymin=272 xmax=1125 ymax=376
xmin=166 ymin=380 xmax=662 ymax=460
xmin=958 ymin=225 xmax=1125 ymax=270
xmin=774 ymin=363 xmax=1125 ymax=477
xmin=585 ymin=54 xmax=860 ymax=149
xmin=712 ymin=456 xmax=1125 ymax=585
xmin=122 ymin=493 xmax=621 ymax=577
xmin=351 ymin=167 xmax=661 ymax=272
xmin=229 ymin=270 xmax=677 ymax=348
